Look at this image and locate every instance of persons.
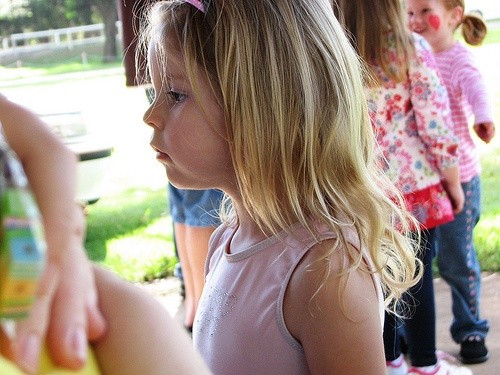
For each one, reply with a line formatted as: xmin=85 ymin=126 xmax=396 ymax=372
xmin=405 ymin=0 xmax=497 ymax=365
xmin=331 ymin=0 xmax=473 ymax=375
xmin=168 ymin=183 xmax=237 ymax=333
xmin=132 ymin=0 xmax=424 ymax=375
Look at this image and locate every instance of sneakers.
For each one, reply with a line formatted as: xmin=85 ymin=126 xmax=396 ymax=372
xmin=460 ymin=335 xmax=489 ymax=364
xmin=387 ymin=349 xmax=472 ymax=375
